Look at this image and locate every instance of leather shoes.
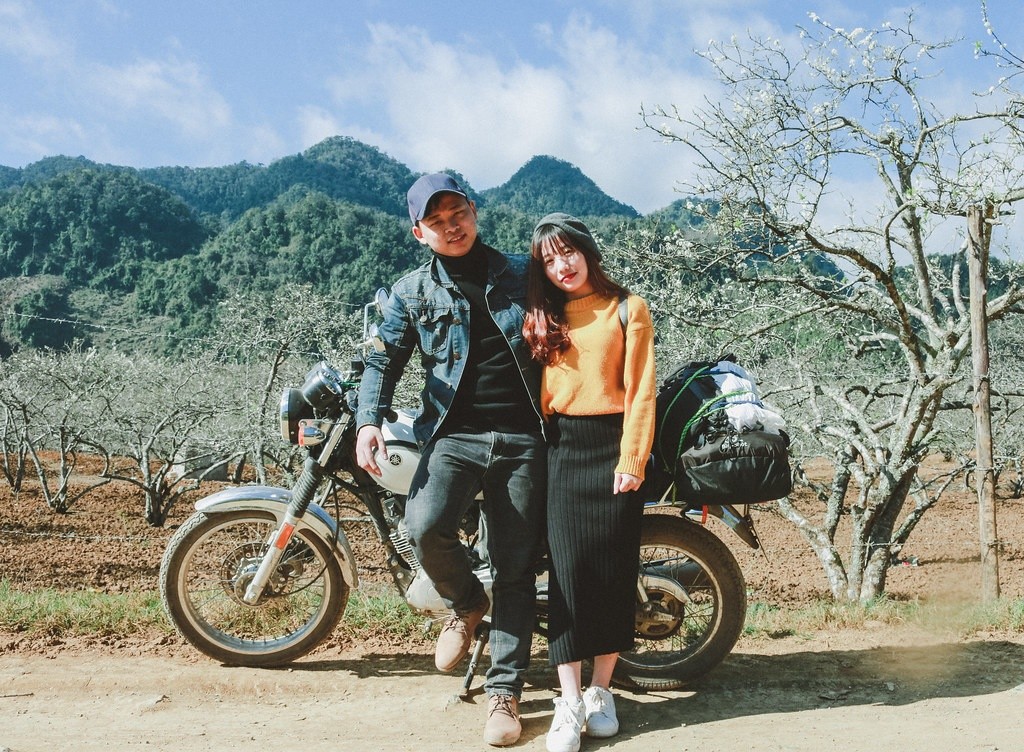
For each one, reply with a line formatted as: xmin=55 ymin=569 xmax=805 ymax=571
xmin=435 ymin=598 xmax=489 ymax=671
xmin=485 ymin=694 xmax=523 ymax=745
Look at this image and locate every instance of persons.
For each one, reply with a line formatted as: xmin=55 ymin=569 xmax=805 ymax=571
xmin=520 ymin=211 xmax=657 ymax=752
xmin=356 ymin=173 xmax=567 ymax=747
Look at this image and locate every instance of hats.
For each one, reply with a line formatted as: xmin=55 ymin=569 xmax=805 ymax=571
xmin=533 ymin=212 xmax=602 ymax=261
xmin=408 ymin=173 xmax=467 ymax=225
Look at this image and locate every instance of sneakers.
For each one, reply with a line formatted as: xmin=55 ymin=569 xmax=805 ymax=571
xmin=545 ymin=685 xmax=619 ymax=752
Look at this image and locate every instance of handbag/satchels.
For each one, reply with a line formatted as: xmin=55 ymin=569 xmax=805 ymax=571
xmin=652 ymin=352 xmax=792 ymax=509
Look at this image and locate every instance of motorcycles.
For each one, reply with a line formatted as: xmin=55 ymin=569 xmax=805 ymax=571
xmin=156 ymin=287 xmax=770 ymax=692
